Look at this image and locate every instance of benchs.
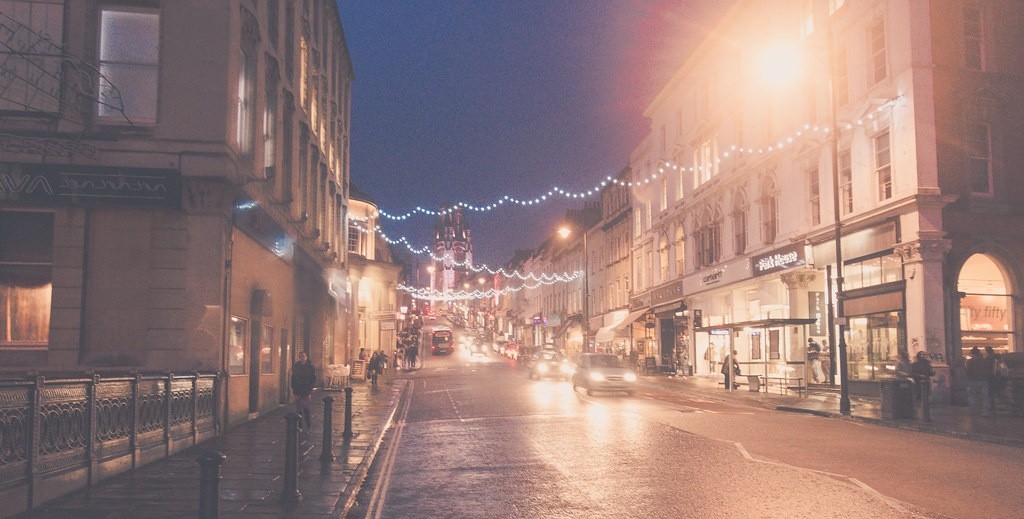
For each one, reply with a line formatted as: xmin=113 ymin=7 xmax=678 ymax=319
xmin=760 ymin=376 xmax=804 ymax=398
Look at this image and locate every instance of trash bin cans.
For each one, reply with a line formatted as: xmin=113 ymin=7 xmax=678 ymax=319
xmin=879 ymin=378 xmax=914 ymax=420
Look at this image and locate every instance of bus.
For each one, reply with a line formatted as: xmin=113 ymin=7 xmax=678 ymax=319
xmin=432 ymin=326 xmax=454 ymax=355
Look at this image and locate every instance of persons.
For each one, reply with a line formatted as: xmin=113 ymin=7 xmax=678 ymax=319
xmin=396 ymin=324 xmax=420 ymax=370
xmin=359 ymin=348 xmax=365 ymax=359
xmin=366 ymin=352 xmax=383 ymax=387
xmin=808 ymin=337 xmax=834 ymax=384
xmin=290 ymin=351 xmax=316 ymax=433
xmin=721 ymin=350 xmax=741 ymax=389
xmin=895 ymin=344 xmax=1009 ymax=418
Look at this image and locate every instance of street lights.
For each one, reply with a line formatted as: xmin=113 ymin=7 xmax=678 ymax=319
xmin=753 ymin=38 xmax=852 ymax=418
xmin=557 ymin=226 xmax=589 ymax=353
xmin=397 ymin=306 xmax=408 ymax=333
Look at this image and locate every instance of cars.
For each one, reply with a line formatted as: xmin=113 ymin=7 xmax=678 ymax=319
xmin=571 ymin=353 xmax=639 ymax=397
xmin=429 ymin=308 xmax=466 ymax=326
xmin=529 ymin=349 xmax=570 ymax=382
xmin=456 ymin=325 xmax=531 ymax=364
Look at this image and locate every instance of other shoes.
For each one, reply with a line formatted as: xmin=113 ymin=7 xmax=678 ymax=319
xmin=298 ymin=422 xmax=311 ymax=429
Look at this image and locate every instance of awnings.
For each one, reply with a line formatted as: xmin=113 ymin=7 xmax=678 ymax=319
xmin=609 ymin=308 xmax=649 ymax=330
